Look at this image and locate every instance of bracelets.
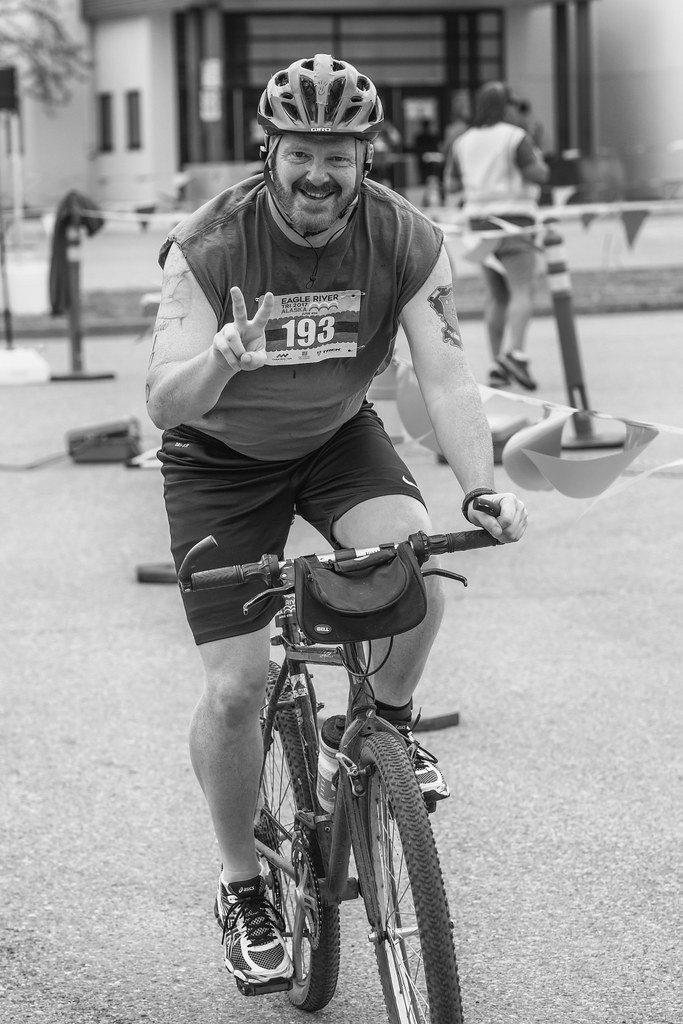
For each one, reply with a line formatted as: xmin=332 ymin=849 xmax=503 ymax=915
xmin=461 ymin=487 xmax=498 ymax=523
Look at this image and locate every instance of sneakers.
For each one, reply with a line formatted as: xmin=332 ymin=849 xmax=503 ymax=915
xmin=374 ymin=695 xmax=450 ymax=801
xmin=213 ymin=862 xmax=294 ymax=984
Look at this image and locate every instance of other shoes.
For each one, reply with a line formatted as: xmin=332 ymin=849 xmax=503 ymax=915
xmin=489 ymin=370 xmax=510 ymax=388
xmin=497 ymin=351 xmax=535 ymax=390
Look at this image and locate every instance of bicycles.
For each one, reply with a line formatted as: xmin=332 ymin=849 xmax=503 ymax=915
xmin=134 ymin=498 xmax=512 ymax=1024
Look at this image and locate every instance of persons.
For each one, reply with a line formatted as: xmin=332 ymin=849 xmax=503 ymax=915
xmin=145 ymin=54 xmax=532 ymax=988
xmin=414 ymin=81 xmax=543 ymax=393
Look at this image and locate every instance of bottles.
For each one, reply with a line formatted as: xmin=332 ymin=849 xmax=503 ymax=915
xmin=315 ymin=714 xmax=350 ymax=814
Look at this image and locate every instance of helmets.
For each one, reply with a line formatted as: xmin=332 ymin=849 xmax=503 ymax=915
xmin=257 ymin=53 xmax=384 ymax=142
